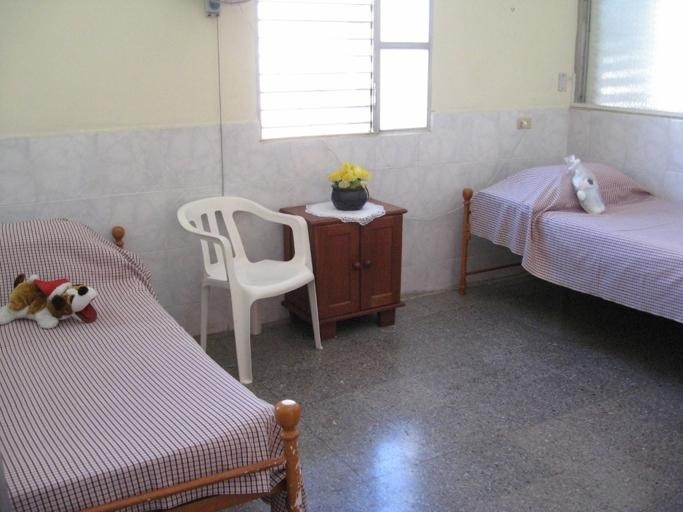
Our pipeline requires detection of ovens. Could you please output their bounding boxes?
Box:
[0,217,305,511]
[456,187,682,337]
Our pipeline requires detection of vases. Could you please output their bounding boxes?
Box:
[327,162,372,190]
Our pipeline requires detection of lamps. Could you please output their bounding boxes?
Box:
[175,195,324,384]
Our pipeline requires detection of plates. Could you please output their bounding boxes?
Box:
[280,199,409,340]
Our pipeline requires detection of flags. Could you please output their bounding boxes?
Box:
[330,186,367,210]
